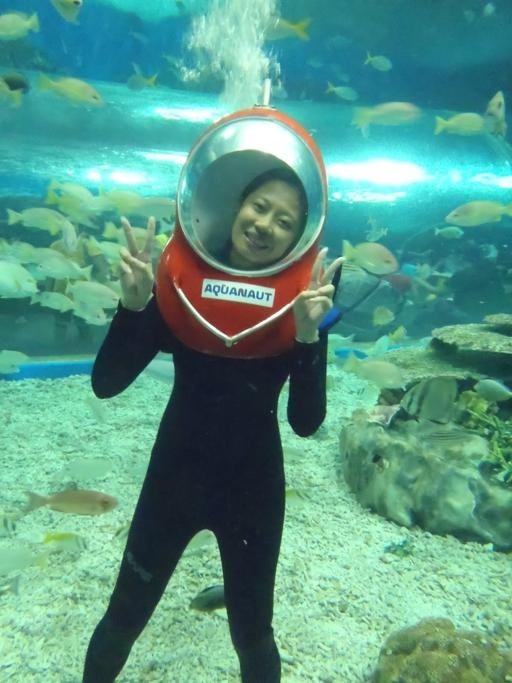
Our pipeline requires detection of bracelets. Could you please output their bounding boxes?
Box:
[117,297,147,320]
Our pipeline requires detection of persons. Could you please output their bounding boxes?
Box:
[83,168,346,682]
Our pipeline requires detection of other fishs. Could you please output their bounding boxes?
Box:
[0,1,512,614]
